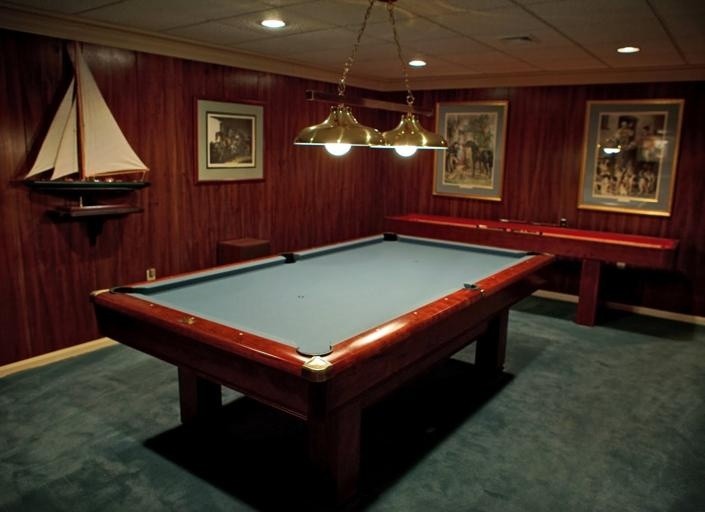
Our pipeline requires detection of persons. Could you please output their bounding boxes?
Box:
[447,141,464,174]
[596,119,658,197]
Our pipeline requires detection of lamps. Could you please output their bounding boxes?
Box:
[288,0,453,160]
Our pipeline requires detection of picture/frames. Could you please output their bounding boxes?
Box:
[189,91,268,188]
[576,97,688,219]
[428,96,510,203]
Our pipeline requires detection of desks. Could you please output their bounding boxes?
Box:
[85,224,560,508]
[386,211,683,329]
